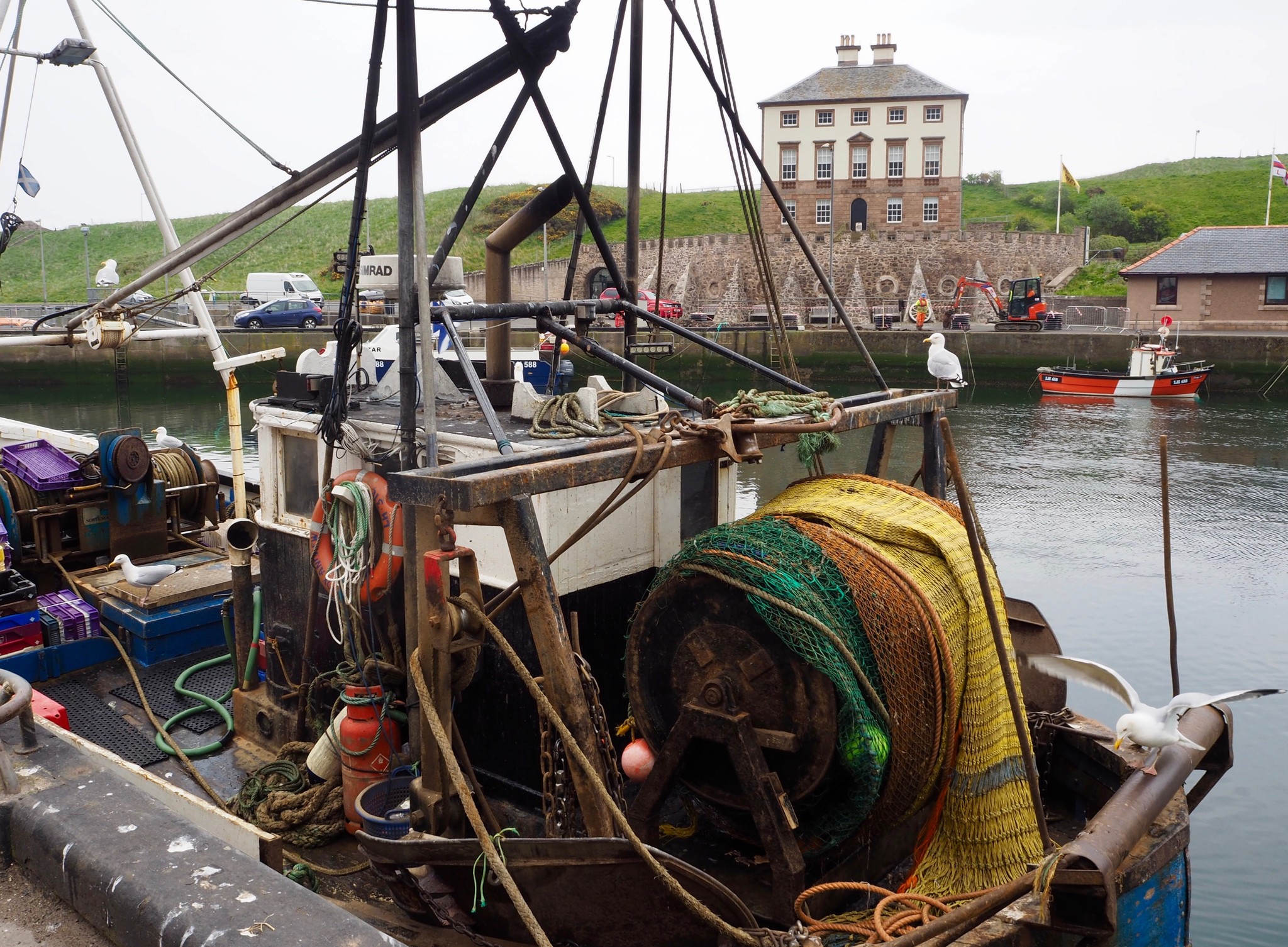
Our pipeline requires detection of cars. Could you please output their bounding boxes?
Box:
[538,325,576,379]
[358,289,386,302]
[239,292,248,304]
[434,285,475,306]
[233,297,325,328]
[113,287,154,304]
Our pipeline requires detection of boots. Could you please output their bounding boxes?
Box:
[916,326,919,331]
[919,325,923,330]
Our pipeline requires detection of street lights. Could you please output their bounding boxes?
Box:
[80,223,91,288]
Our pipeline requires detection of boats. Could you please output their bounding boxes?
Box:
[1037,312,1213,399]
[348,315,573,391]
[0,0,1235,947]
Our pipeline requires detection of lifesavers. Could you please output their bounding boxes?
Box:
[1157,326,1170,337]
[1140,344,1164,351]
[309,470,404,605]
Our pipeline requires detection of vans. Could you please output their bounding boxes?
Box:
[246,272,325,309]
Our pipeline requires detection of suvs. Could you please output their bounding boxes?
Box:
[599,287,683,321]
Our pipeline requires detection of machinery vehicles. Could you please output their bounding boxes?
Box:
[943,276,1048,332]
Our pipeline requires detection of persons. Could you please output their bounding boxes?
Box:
[914,294,929,331]
[592,286,601,299]
[1027,285,1035,298]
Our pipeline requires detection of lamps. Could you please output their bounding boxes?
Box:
[625,333,675,356]
[48,38,95,66]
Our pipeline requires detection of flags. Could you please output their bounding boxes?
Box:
[1273,156,1288,186]
[1061,163,1080,194]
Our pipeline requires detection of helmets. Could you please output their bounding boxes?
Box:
[921,293,926,297]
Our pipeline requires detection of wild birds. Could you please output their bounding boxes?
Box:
[923,333,968,391]
[1028,653,1288,776]
[108,554,185,607]
[151,426,199,453]
[96,259,119,298]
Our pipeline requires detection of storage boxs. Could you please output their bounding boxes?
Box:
[0,569,101,658]
[2,439,84,492]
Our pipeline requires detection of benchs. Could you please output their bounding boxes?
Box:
[748,305,775,322]
[872,305,902,323]
[809,306,838,324]
[699,303,719,315]
